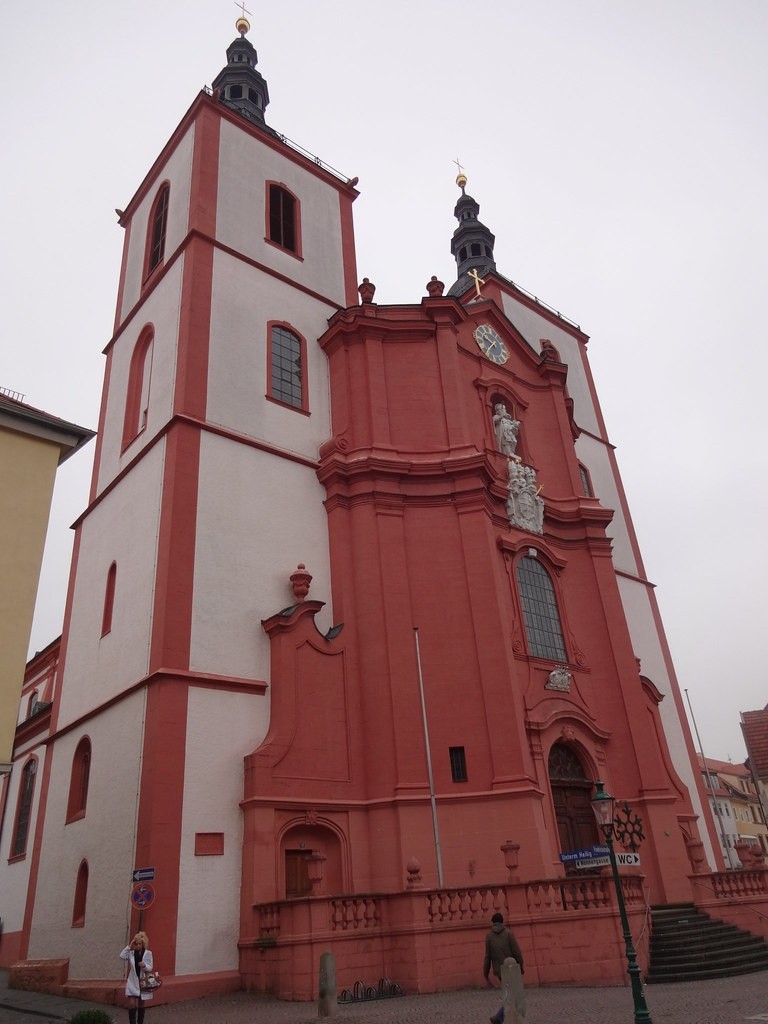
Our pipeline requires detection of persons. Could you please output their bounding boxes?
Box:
[120,932,155,1024]
[507,461,537,492]
[493,404,520,455]
[483,913,526,1024]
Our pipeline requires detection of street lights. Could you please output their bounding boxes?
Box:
[589,780,652,1024]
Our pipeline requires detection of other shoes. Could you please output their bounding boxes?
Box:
[490,1014,501,1024]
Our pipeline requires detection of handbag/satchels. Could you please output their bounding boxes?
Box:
[139,968,163,992]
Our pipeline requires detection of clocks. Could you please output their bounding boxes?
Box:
[473,324,511,366]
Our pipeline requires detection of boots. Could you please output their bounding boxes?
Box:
[137,1007,145,1024]
[128,1006,137,1024]
